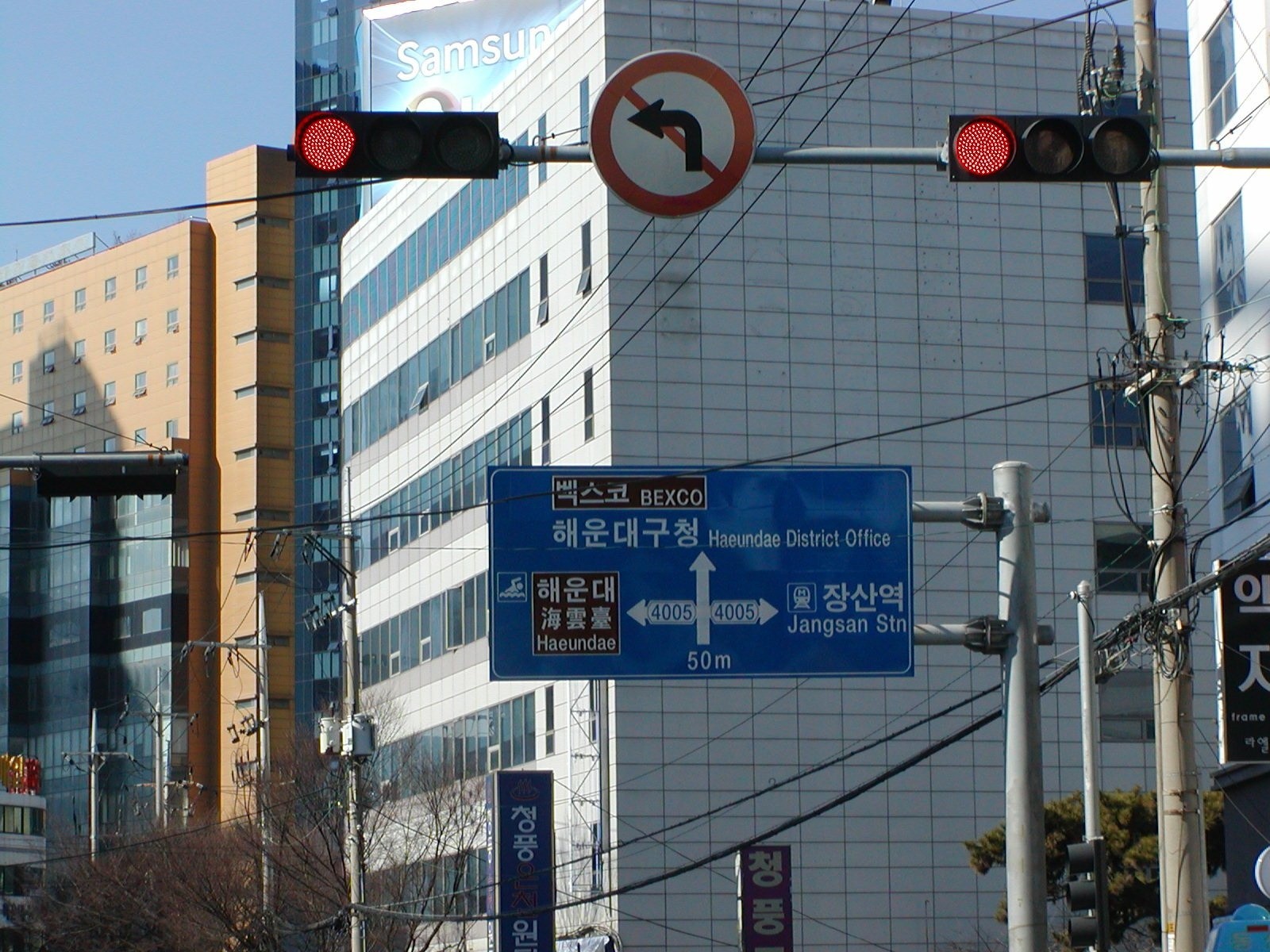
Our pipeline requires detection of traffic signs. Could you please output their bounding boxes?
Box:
[485,463,916,680]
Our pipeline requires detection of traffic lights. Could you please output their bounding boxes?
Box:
[1064,837,1111,952]
[949,114,1153,185]
[293,110,500,181]
